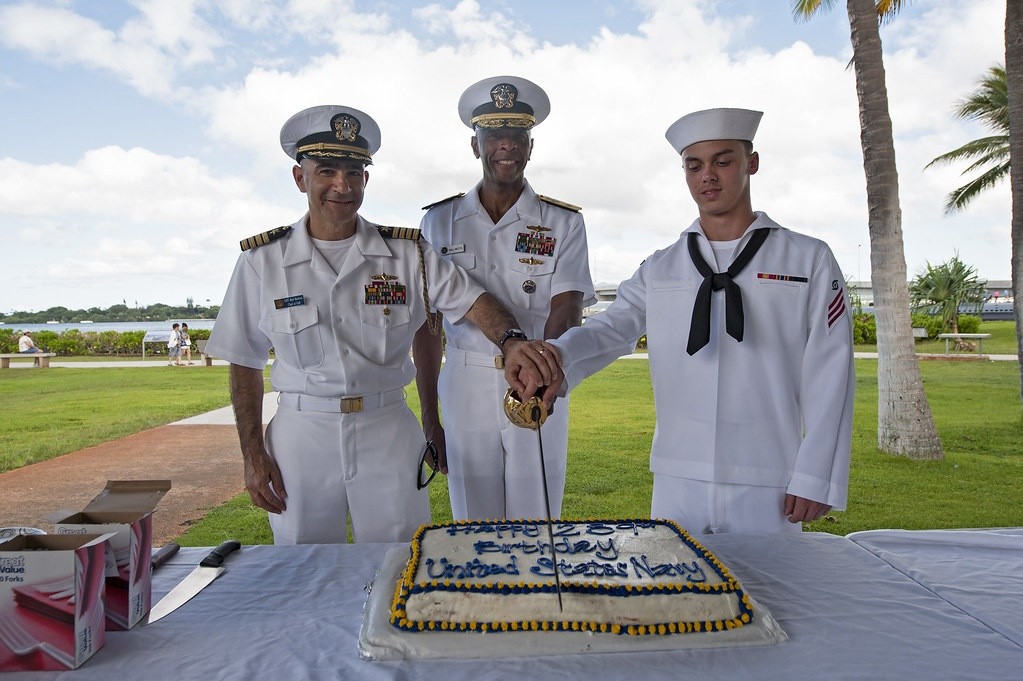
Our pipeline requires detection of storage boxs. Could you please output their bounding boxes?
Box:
[0,528,121,673]
[53,477,173,633]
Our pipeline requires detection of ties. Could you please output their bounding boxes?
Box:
[686,228,770,356]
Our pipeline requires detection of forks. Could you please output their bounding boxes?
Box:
[115,548,130,571]
[33,576,75,604]
[0,613,75,669]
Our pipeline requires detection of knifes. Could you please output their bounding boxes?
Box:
[140,539,241,626]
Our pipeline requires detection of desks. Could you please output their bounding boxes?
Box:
[0,532,1023,681]
[845,506,1023,645]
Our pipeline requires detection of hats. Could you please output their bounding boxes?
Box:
[459,76,550,130]
[666,109,764,154]
[23,329,33,334]
[280,105,381,165]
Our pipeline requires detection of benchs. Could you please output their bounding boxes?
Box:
[0,352,57,369]
[939,333,992,355]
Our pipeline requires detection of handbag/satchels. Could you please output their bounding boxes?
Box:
[185,338,191,346]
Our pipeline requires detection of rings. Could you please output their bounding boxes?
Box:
[539,348,547,355]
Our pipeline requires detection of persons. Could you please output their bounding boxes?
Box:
[517,108,857,533]
[19,328,43,366]
[203,104,563,544]
[175,323,195,365]
[168,322,184,366]
[412,77,598,522]
[994,290,999,303]
[1004,290,1008,299]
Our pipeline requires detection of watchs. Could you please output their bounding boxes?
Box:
[499,329,527,347]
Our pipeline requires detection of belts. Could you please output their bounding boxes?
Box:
[446,347,504,370]
[280,389,404,414]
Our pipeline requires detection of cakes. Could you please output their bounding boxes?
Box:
[390,516,755,636]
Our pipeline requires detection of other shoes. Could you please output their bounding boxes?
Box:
[169,363,173,366]
[188,362,194,366]
[177,363,185,366]
